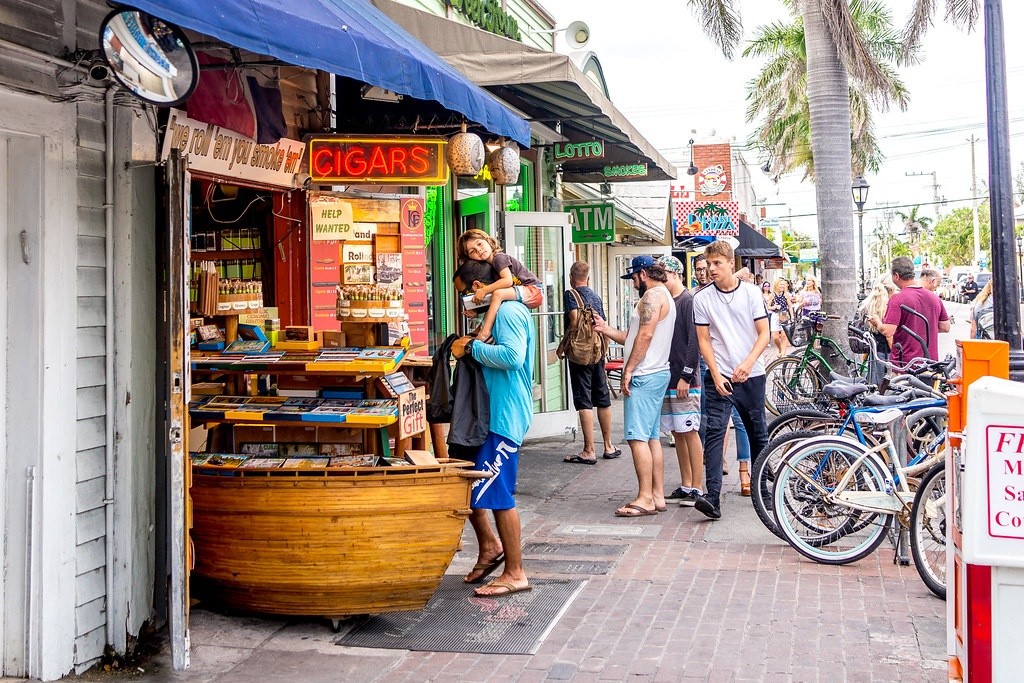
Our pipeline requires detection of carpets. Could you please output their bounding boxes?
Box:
[334,575,588,656]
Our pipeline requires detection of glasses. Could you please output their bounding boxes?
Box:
[763,286,770,288]
[626,266,646,274]
[458,279,482,299]
[696,267,707,274]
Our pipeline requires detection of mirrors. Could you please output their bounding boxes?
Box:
[99,7,199,107]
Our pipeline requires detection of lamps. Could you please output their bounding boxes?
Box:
[361,84,404,103]
[687,138,698,175]
[529,21,590,49]
[730,141,779,183]
[484,138,506,153]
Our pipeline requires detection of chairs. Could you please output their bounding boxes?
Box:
[603,353,625,393]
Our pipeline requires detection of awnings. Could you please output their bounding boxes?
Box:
[734,219,783,257]
[372,0,676,181]
[98,0,532,151]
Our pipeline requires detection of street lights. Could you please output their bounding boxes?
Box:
[851,176,869,300]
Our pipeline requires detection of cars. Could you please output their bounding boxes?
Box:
[939,266,992,303]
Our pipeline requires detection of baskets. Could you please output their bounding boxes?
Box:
[772,373,831,417]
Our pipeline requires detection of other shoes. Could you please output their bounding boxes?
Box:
[722,458,729,475]
[702,451,707,464]
[669,433,676,447]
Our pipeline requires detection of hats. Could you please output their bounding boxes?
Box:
[620,255,657,279]
[655,255,684,273]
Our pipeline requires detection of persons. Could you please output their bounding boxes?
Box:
[964,277,994,340]
[916,268,955,325]
[556,261,622,464]
[694,241,773,519]
[589,256,676,516]
[962,275,978,302]
[867,273,895,384]
[756,274,821,357]
[653,257,704,506]
[451,260,535,596]
[707,267,751,495]
[459,228,543,346]
[691,254,707,465]
[869,256,951,479]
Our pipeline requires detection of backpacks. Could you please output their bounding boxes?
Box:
[563,290,605,365]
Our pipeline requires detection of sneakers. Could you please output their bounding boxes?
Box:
[694,495,721,518]
[664,488,689,504]
[680,489,704,506]
[760,486,773,510]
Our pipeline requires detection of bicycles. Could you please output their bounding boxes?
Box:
[751,309,959,599]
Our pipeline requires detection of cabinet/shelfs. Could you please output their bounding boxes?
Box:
[189,360,421,458]
[190,250,262,312]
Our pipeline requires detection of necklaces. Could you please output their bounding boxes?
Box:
[716,275,735,304]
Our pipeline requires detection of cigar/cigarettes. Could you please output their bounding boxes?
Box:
[196,258,262,317]
[336,285,404,300]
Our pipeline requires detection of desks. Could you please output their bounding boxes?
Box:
[607,344,625,393]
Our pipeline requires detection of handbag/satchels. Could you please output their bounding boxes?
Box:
[779,315,789,326]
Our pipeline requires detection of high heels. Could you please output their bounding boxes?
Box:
[739,469,752,496]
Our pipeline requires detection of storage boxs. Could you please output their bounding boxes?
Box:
[190,318,407,372]
[190,370,415,425]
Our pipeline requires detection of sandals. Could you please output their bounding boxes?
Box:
[468,332,478,337]
[482,334,495,345]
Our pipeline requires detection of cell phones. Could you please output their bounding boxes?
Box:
[724,382,732,392]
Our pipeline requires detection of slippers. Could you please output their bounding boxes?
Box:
[655,505,667,512]
[466,555,505,583]
[475,577,533,597]
[603,446,622,458]
[563,454,597,464]
[615,503,659,516]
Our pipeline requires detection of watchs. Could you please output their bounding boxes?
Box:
[464,339,475,355]
